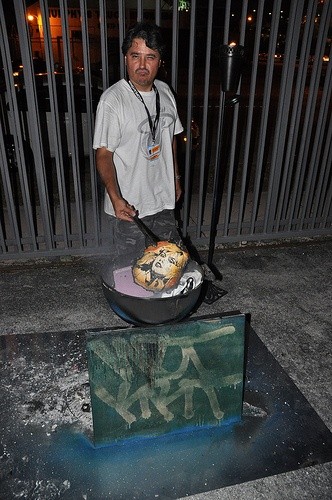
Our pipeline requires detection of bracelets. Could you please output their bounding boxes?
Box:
[175,176,180,178]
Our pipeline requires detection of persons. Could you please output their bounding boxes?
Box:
[93,21,188,271]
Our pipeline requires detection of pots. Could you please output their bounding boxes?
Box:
[100,254,202,326]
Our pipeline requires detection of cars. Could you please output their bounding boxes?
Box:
[0,81,208,175]
